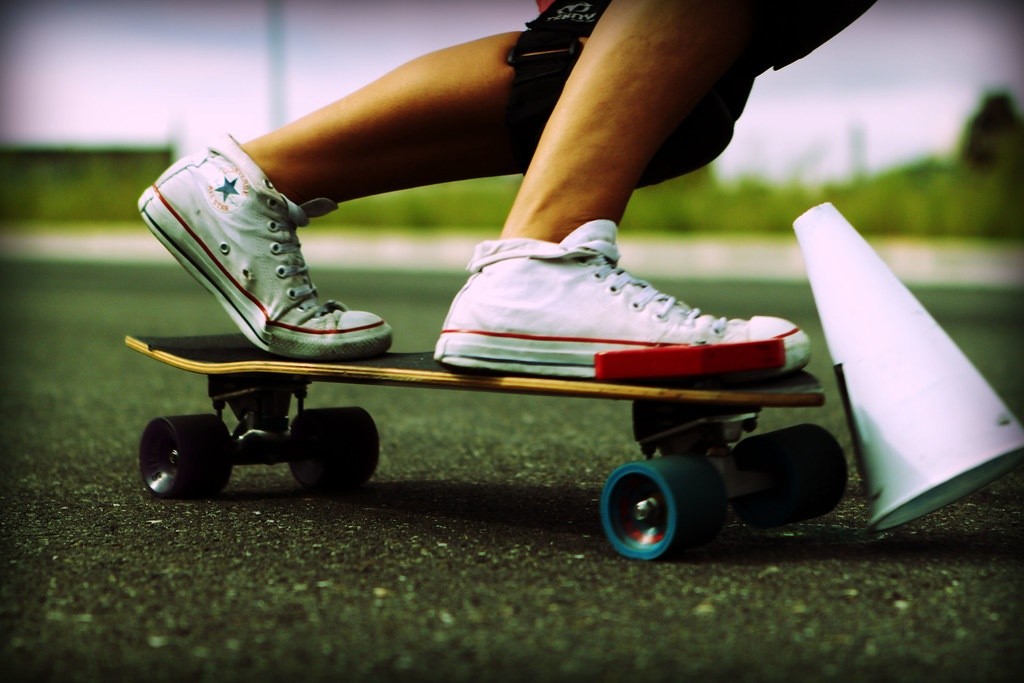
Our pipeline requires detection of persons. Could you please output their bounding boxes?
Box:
[130,0,905,388]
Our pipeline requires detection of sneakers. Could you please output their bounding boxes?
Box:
[137,132,394,361]
[432,218,812,382]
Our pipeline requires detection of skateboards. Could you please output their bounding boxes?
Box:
[124,330,849,559]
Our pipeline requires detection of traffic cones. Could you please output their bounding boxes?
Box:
[791,200,1023,533]
